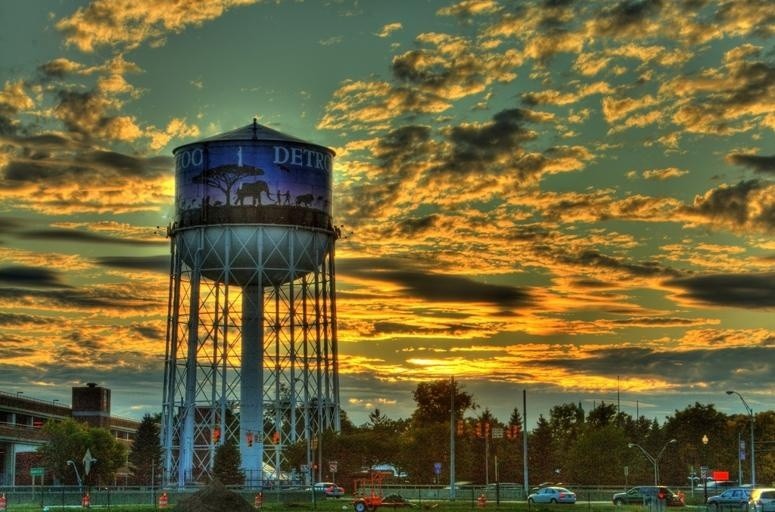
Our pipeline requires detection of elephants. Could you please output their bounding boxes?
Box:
[233,179,275,206]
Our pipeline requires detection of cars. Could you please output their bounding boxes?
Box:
[748,487,775,512]
[304,481,345,500]
[527,487,577,505]
[612,486,684,506]
[706,487,752,512]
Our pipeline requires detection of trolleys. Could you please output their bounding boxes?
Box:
[350,478,413,512]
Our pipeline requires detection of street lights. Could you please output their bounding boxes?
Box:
[725,390,757,486]
[627,439,678,486]
